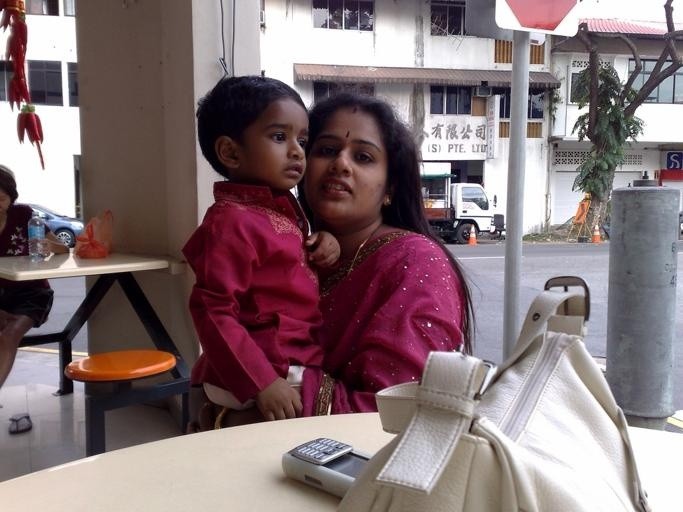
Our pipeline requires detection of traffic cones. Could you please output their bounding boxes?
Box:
[468,224,477,246]
[591,225,600,242]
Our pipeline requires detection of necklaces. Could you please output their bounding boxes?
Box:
[345,233,375,279]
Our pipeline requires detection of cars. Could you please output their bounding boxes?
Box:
[17,204,85,248]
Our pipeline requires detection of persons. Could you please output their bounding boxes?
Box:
[0,162,69,410]
[178,75,342,423]
[186,85,481,434]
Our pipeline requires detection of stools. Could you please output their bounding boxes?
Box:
[62,348,177,462]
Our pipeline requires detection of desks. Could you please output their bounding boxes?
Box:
[1,244,191,434]
[1,411,683,510]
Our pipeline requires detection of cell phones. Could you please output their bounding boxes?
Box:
[283,435,372,500]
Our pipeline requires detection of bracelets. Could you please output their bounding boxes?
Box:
[214,406,227,432]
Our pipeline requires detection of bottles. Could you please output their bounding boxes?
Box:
[27,211,46,264]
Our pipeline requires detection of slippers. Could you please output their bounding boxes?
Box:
[8,412,32,433]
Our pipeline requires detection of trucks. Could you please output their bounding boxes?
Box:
[419,173,507,245]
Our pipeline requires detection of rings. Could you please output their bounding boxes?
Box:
[42,253,47,257]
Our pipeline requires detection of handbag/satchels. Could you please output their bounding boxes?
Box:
[336,276,650,511]
